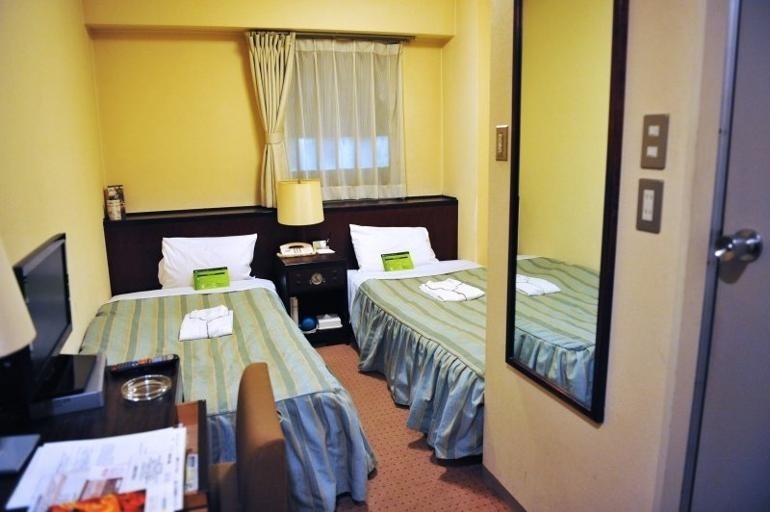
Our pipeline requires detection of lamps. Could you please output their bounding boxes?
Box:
[277,179,326,226]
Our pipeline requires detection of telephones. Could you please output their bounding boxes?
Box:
[279,241,315,257]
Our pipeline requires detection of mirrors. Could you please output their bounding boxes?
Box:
[503,0,629,425]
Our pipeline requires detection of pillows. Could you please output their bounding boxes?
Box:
[158,234,257,291]
[349,224,439,272]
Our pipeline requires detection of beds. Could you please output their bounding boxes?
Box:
[346,221,486,460]
[515,256,599,412]
[80,237,378,511]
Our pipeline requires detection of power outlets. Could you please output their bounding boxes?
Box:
[634,176,664,234]
[494,125,508,163]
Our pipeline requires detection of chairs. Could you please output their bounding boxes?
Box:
[213,362,293,511]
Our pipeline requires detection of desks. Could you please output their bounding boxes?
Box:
[0,354,205,512]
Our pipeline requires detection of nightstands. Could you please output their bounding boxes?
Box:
[275,244,352,345]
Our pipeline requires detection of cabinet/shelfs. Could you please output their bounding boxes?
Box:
[103,194,458,346]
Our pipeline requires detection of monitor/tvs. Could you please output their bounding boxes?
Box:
[14,231,98,401]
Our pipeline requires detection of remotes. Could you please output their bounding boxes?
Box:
[109,352,180,377]
